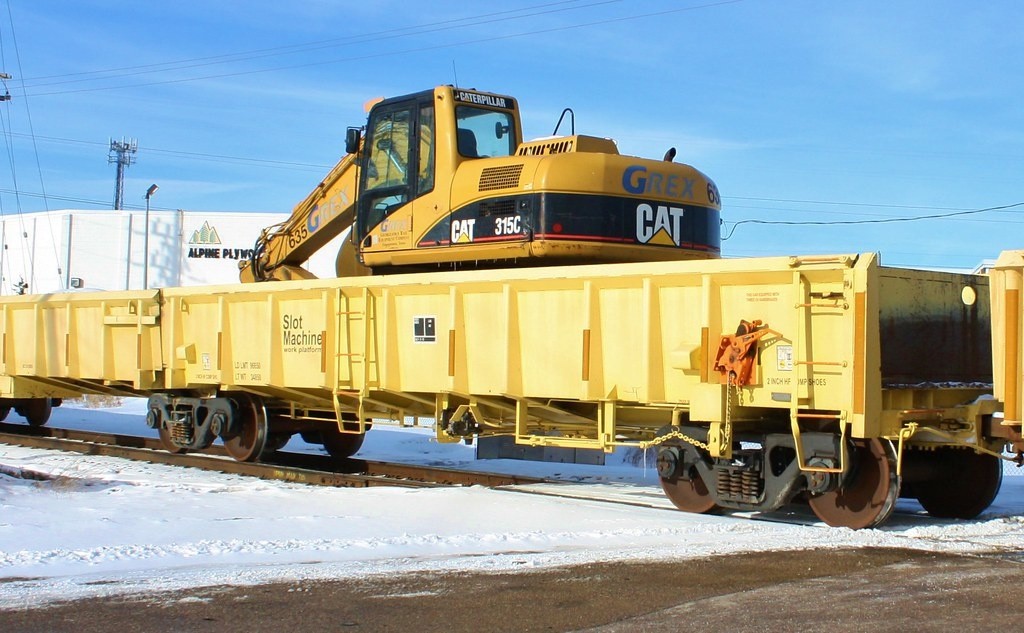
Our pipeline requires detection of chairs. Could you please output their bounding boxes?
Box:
[456,128,477,158]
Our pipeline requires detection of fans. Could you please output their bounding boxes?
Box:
[496,122,509,138]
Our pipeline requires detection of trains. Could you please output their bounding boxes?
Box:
[1,249,1023,531]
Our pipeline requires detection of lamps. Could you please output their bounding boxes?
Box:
[71,278,80,287]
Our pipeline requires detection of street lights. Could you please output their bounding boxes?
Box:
[144,184,160,290]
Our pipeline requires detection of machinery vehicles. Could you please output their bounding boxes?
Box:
[237,84,722,283]
